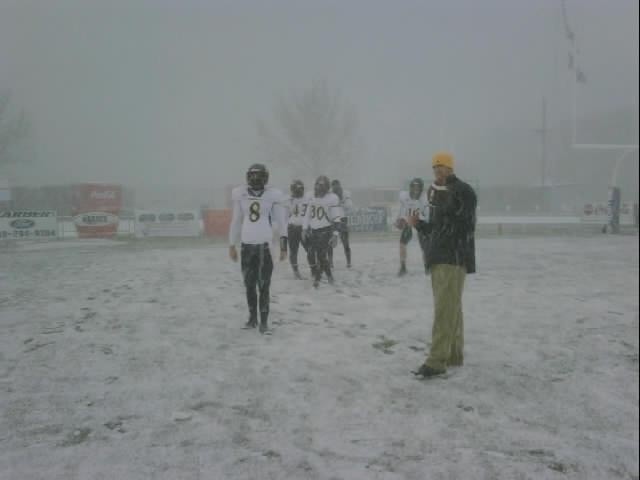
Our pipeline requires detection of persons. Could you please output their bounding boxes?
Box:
[286,180,317,280]
[301,175,341,286]
[327,179,352,269]
[227,161,292,336]
[409,152,477,381]
[609,185,621,234]
[392,177,430,278]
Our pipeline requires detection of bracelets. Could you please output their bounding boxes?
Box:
[280,235,288,251]
[332,230,339,235]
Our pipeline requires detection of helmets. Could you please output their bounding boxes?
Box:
[432,153,453,169]
[314,176,330,197]
[247,163,269,188]
[409,178,424,199]
[290,180,304,197]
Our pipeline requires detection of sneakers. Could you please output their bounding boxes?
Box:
[293,270,334,287]
[397,265,407,276]
[411,363,448,378]
[259,320,272,334]
[241,318,258,329]
